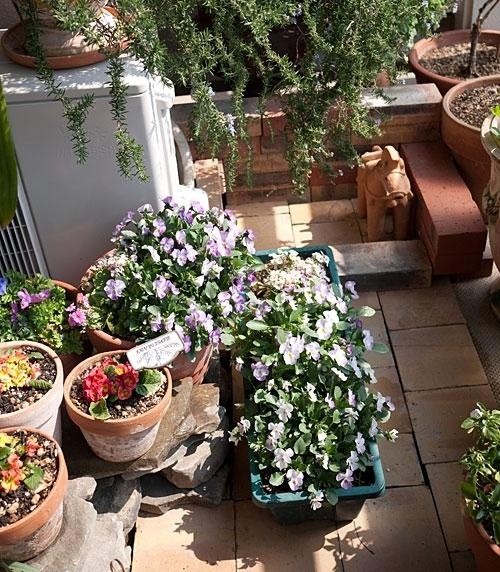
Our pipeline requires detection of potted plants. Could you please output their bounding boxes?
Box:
[460,402,500,572]
[0,0,449,197]
[480,105,499,319]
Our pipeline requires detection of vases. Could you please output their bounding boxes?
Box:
[244,245,386,525]
[82,248,212,385]
[0,426,68,562]
[0,341,64,448]
[409,27,500,95]
[64,350,172,463]
[50,279,93,380]
[442,74,500,224]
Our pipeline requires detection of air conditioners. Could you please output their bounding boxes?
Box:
[0,54,193,287]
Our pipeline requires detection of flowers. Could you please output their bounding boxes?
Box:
[0,346,53,394]
[0,270,103,356]
[229,251,399,511]
[90,196,272,362]
[0,433,44,493]
[82,357,166,420]
